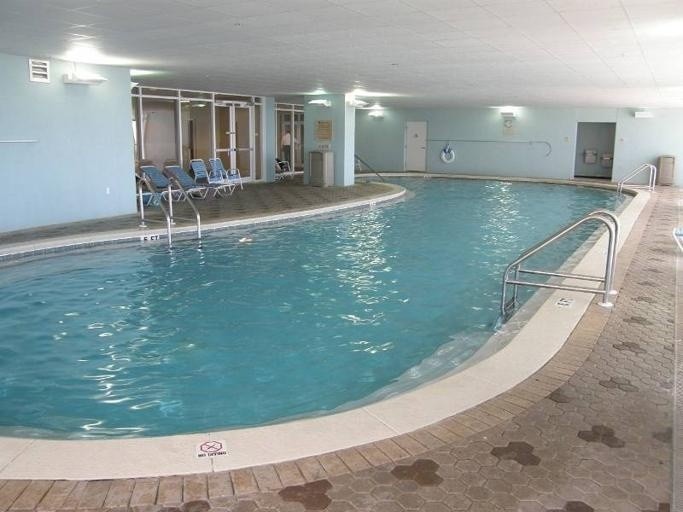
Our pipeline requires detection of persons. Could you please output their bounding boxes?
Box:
[280,130,300,168]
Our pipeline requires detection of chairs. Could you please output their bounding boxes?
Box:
[139,158,244,207]
[272,160,294,182]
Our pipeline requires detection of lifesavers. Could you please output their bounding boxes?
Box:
[441,148,454,162]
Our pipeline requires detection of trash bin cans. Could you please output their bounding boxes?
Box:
[309,151,335,188]
[655,155,675,186]
